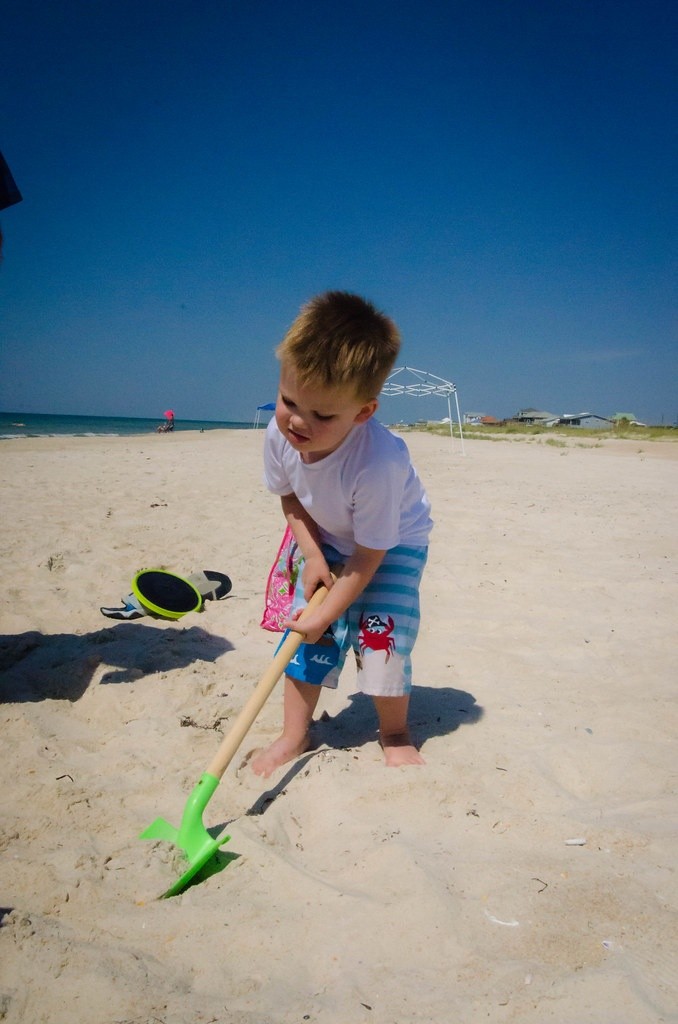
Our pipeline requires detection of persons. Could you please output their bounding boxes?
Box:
[169,414,175,431]
[249,290,434,777]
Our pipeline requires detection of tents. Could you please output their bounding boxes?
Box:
[252,402,277,429]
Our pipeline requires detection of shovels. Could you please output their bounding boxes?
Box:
[140,563,345,899]
